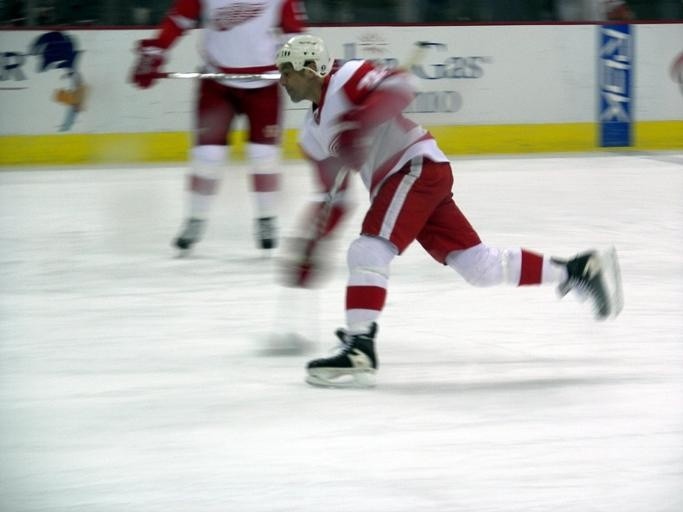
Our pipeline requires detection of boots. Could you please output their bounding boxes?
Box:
[175,217,205,249]
[257,219,277,249]
[306,323,378,377]
[551,249,613,323]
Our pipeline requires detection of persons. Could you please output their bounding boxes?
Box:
[128,1,306,251]
[0,0,672,32]
[274,34,614,378]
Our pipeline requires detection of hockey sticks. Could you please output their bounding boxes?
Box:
[153,41,429,79]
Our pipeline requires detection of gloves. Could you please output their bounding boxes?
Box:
[327,110,368,170]
[125,39,165,91]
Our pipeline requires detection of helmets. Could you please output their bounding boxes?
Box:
[275,36,334,78]
